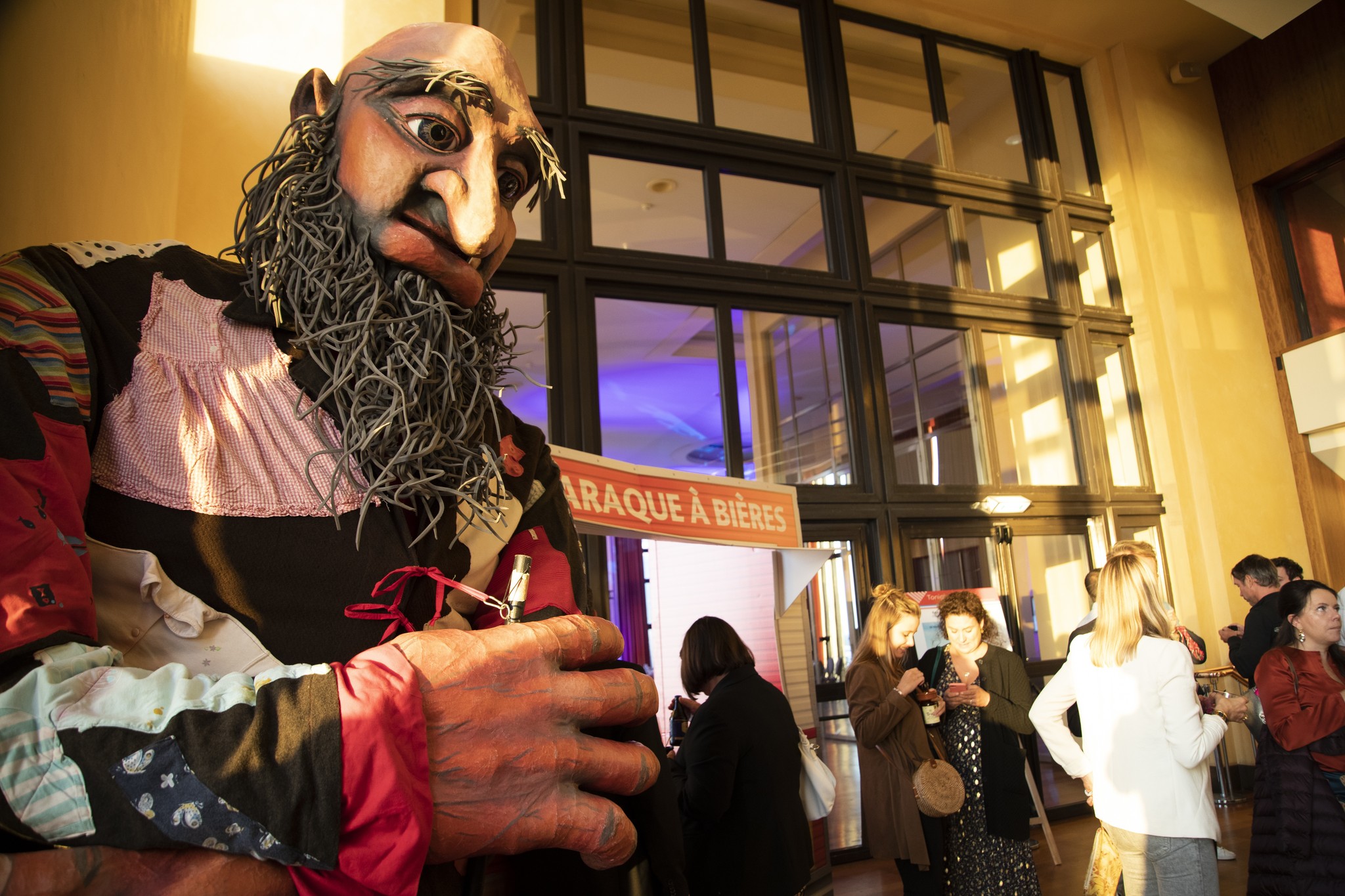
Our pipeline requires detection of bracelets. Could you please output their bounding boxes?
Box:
[1209,710,1229,726]
[893,686,906,699]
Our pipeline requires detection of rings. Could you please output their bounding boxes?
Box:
[970,698,973,704]
[1242,713,1248,720]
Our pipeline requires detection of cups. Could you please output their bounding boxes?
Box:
[917,690,942,727]
[1210,689,1241,709]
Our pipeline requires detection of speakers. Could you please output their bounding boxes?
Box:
[1169,62,1202,84]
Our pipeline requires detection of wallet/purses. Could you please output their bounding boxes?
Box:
[1083,820,1122,896]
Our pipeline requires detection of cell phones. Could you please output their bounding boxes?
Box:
[949,683,968,695]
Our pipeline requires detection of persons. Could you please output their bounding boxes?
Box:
[1067,538,1207,737]
[1029,553,1250,895]
[1217,554,1284,689]
[2,21,661,894]
[846,582,950,896]
[666,617,815,895]
[1269,558,1303,589]
[916,592,1044,896]
[1246,580,1345,896]
[1074,567,1179,627]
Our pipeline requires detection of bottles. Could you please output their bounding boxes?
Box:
[669,695,689,746]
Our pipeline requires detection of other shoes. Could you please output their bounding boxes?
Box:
[1217,846,1237,860]
[1028,837,1039,849]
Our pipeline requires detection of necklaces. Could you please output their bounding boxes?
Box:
[963,671,971,678]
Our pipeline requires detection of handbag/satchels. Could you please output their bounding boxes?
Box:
[913,758,965,818]
[798,725,836,821]
[1239,655,1298,744]
